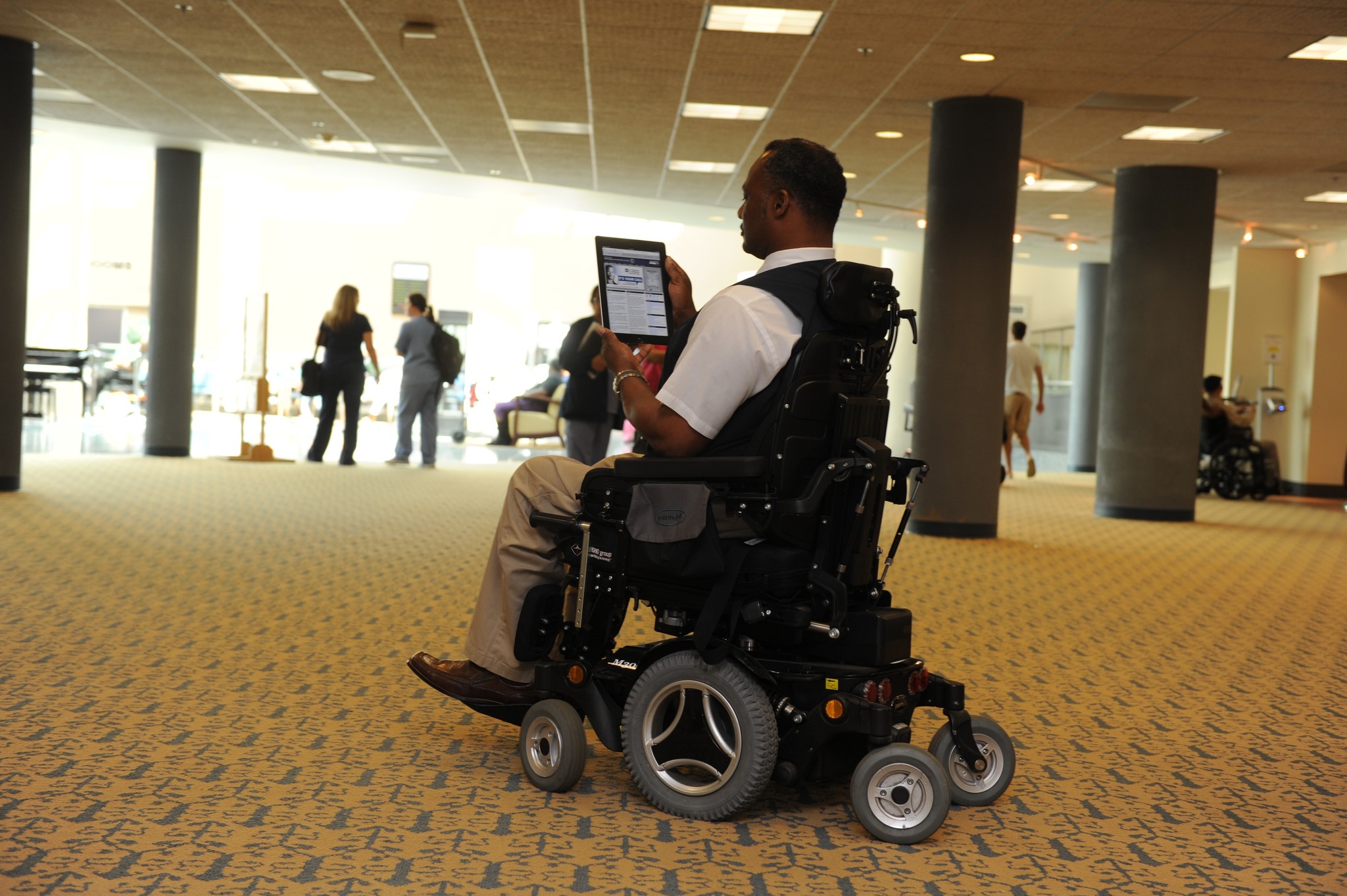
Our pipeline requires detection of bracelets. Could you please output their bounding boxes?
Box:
[612,370,650,401]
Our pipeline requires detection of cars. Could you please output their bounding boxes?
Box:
[435,384,469,443]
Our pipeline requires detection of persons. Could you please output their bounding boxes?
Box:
[559,285,619,464]
[489,358,560,445]
[1004,322,1046,479]
[384,293,443,469]
[606,265,617,284]
[403,138,849,707]
[307,286,382,466]
[1201,374,1283,501]
[85,340,149,407]
[632,341,669,457]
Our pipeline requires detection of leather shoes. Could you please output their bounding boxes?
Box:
[406,651,547,708]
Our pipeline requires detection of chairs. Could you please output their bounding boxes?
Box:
[506,382,569,451]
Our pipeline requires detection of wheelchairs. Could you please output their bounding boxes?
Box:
[1199,398,1276,504]
[508,252,1022,846]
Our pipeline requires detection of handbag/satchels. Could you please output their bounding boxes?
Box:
[301,334,326,397]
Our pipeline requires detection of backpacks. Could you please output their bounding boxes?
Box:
[430,320,465,384]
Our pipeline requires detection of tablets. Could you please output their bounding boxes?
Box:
[594,235,674,346]
